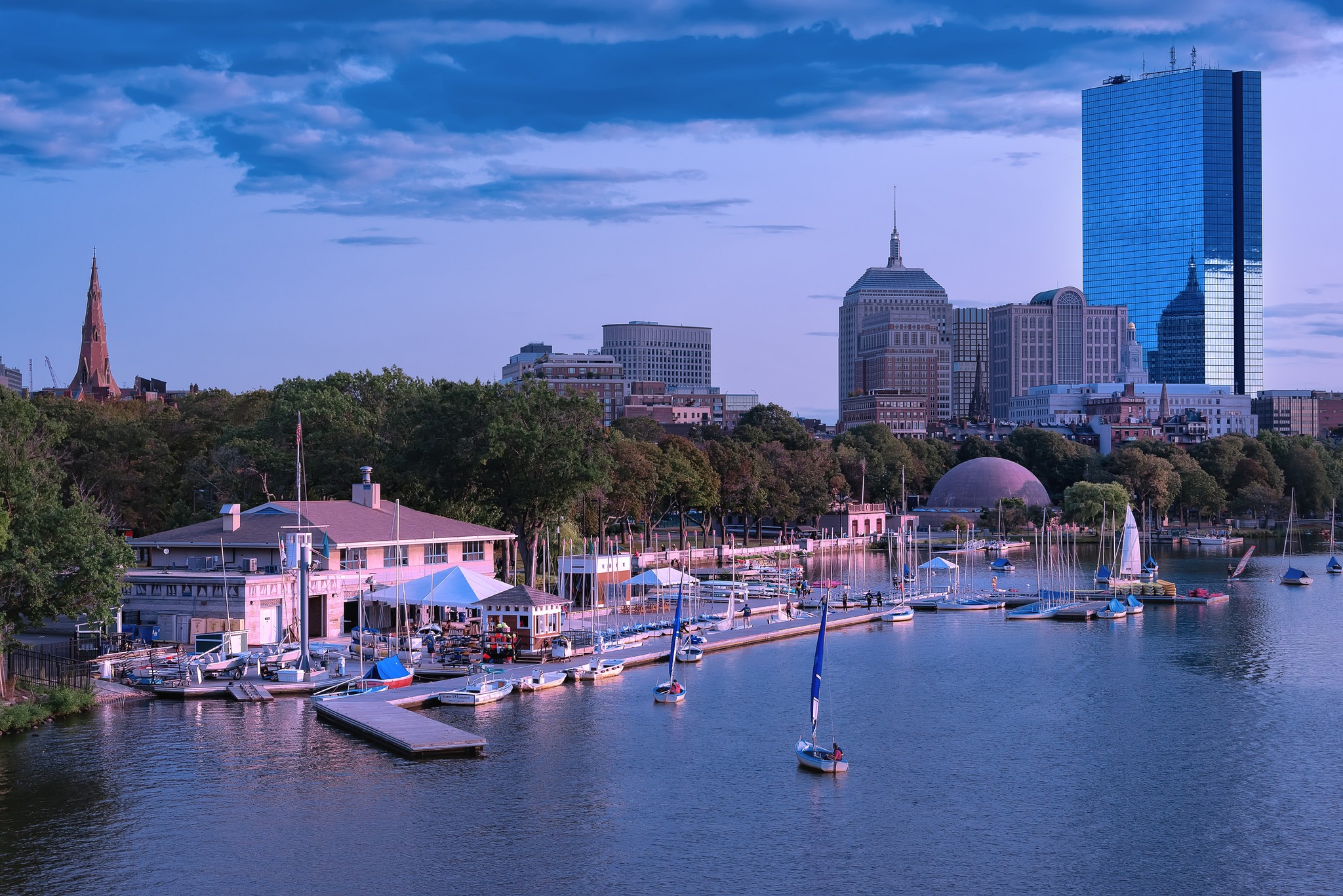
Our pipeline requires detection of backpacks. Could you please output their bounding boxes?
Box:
[801,583,805,589]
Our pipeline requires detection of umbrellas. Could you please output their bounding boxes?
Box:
[607,545,627,554]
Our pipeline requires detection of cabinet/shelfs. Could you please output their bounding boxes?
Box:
[483,631,518,663]
[621,605,647,615]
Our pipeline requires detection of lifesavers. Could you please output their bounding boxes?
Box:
[520,621,524,627]
[601,643,606,652]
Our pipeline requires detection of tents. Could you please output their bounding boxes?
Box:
[917,556,960,594]
[363,566,515,646]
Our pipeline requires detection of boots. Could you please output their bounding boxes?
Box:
[876,606,878,612]
[881,607,884,611]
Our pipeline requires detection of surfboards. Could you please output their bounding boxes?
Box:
[1224,578,1240,581]
[750,567,792,570]
[738,571,810,598]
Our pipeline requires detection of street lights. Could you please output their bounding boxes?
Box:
[1178,486,1182,532]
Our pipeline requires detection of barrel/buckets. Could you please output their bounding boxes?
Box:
[507,657,512,663]
[320,660,330,673]
[491,636,517,643]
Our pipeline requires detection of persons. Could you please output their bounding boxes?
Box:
[736,573,999,629]
[926,528,929,537]
[728,539,736,549]
[445,619,454,633]
[660,544,678,552]
[519,618,524,627]
[1121,574,1136,580]
[908,529,910,534]
[990,533,1028,546]
[1227,564,1237,579]
[777,531,880,546]
[822,743,844,762]
[407,608,414,633]
[422,631,436,664]
[562,604,570,620]
[953,530,956,535]
[669,679,682,695]
[673,558,682,572]
[633,549,639,557]
[416,609,423,630]
[493,555,515,571]
[1079,510,1339,543]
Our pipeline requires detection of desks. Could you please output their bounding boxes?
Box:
[683,597,705,608]
[634,603,657,613]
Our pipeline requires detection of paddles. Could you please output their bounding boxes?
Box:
[435,636,481,663]
[1324,538,1337,541]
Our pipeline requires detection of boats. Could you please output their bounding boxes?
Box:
[509,666,567,692]
[438,662,514,706]
[1320,529,1340,543]
[564,656,627,680]
[1179,534,1244,545]
[1304,533,1311,534]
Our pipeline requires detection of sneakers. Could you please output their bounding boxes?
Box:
[431,661,435,664]
[429,661,431,664]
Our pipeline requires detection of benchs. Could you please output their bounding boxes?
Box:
[620,602,681,615]
[646,602,669,612]
[682,599,707,609]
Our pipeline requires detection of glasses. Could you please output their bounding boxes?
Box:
[843,592,845,592]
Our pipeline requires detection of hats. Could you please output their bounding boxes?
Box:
[895,574,897,577]
[429,631,435,635]
[995,576,997,579]
[1129,575,1131,577]
[672,682,675,686]
[823,594,827,596]
[673,679,677,683]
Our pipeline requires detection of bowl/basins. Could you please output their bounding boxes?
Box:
[111,677,119,682]
[484,658,491,663]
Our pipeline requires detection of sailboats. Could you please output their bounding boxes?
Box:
[881,533,917,622]
[513,497,1177,655]
[794,574,849,773]
[675,542,702,663]
[125,514,443,704]
[1279,487,1314,585]
[1223,545,1255,581]
[652,555,687,704]
[1325,498,1343,573]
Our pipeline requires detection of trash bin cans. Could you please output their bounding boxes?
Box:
[983,529,989,536]
[921,527,925,532]
[121,623,137,640]
[1115,531,1121,536]
[925,527,928,532]
[449,611,458,622]
[459,612,466,622]
[917,525,922,532]
[136,624,162,645]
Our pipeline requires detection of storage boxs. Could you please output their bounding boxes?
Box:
[277,669,305,682]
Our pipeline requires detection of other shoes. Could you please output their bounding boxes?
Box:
[827,617,829,618]
[867,610,871,612]
[803,596,806,599]
[798,597,801,599]
[680,646,683,648]
[846,612,847,613]
[843,611,845,613]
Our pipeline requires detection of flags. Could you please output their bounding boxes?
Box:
[296,414,300,445]
[321,530,329,559]
[280,533,287,565]
[589,539,594,556]
